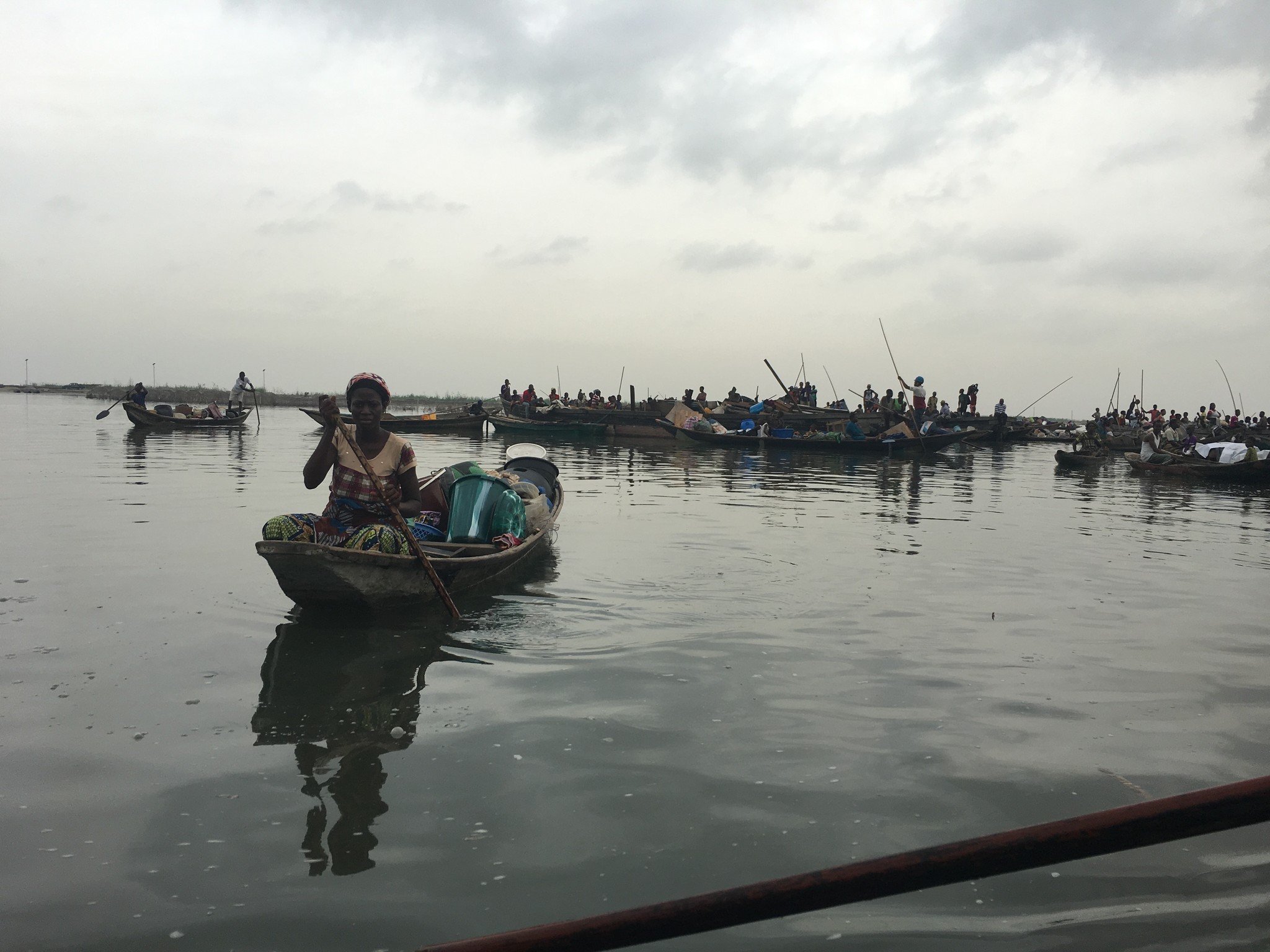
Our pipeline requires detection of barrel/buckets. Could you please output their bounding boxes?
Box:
[1037,432,1045,438]
[706,417,719,424]
[504,456,559,489]
[499,468,553,500]
[446,473,511,546]
[967,426,975,431]
[1028,417,1035,423]
[741,419,755,430]
[506,443,547,466]
[1042,421,1047,426]
[153,405,173,417]
[147,410,157,413]
[218,409,227,416]
[750,400,766,414]
[953,425,961,432]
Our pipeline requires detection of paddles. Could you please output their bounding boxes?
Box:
[95,382,143,420]
[421,772,1270,951]
[319,392,462,620]
[252,388,261,425]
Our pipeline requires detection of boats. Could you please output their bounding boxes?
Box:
[1091,414,1270,476]
[1051,449,1108,468]
[122,401,255,431]
[252,454,564,611]
[486,399,1081,452]
[297,406,503,431]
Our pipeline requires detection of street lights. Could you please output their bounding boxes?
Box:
[262,369,266,393]
[25,359,28,385]
[152,363,156,387]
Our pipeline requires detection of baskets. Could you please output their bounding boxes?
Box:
[411,521,446,543]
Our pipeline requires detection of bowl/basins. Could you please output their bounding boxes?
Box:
[771,429,794,438]
[176,406,193,415]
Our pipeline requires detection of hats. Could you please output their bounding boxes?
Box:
[345,373,391,409]
[976,412,980,415]
[551,387,555,390]
[940,400,945,403]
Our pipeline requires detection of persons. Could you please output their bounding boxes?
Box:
[1230,409,1241,427]
[1067,395,1270,455]
[1206,403,1222,420]
[750,381,981,420]
[501,379,659,428]
[682,389,692,400]
[1244,436,1258,462]
[261,372,422,556]
[1141,404,1160,422]
[457,399,489,420]
[227,372,255,409]
[696,386,707,402]
[1140,419,1173,465]
[126,382,148,410]
[1072,420,1101,454]
[846,412,880,441]
[898,376,926,437]
[994,399,1008,426]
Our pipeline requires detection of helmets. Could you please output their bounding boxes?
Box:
[914,376,924,384]
